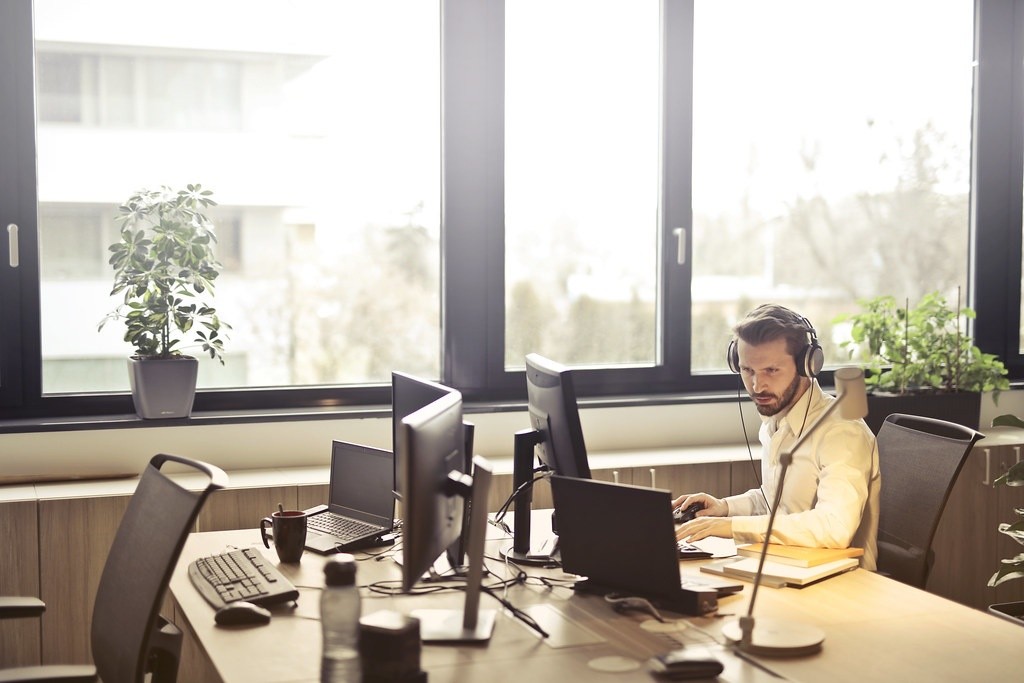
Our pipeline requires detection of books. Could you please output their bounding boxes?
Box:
[699,543,865,589]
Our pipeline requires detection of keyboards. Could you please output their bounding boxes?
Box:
[188,547,299,609]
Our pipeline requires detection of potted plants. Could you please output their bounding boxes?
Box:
[832,292,1011,439]
[93,181,235,420]
[989,412,1024,627]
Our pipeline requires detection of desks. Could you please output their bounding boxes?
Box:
[155,509,1024,683]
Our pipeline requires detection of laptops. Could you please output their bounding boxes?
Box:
[304,440,394,555]
[551,475,744,606]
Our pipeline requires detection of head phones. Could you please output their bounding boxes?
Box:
[727,315,824,377]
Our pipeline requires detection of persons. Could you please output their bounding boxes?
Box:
[671,303,882,573]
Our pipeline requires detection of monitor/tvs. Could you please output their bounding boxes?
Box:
[390,371,475,627]
[499,352,592,563]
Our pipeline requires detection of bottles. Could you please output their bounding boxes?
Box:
[320,555,361,660]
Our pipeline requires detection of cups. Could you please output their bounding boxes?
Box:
[260,511,307,562]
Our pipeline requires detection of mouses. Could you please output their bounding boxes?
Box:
[214,601,271,624]
[672,502,705,523]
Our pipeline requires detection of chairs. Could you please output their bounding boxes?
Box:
[871,411,986,591]
[0,452,231,683]
